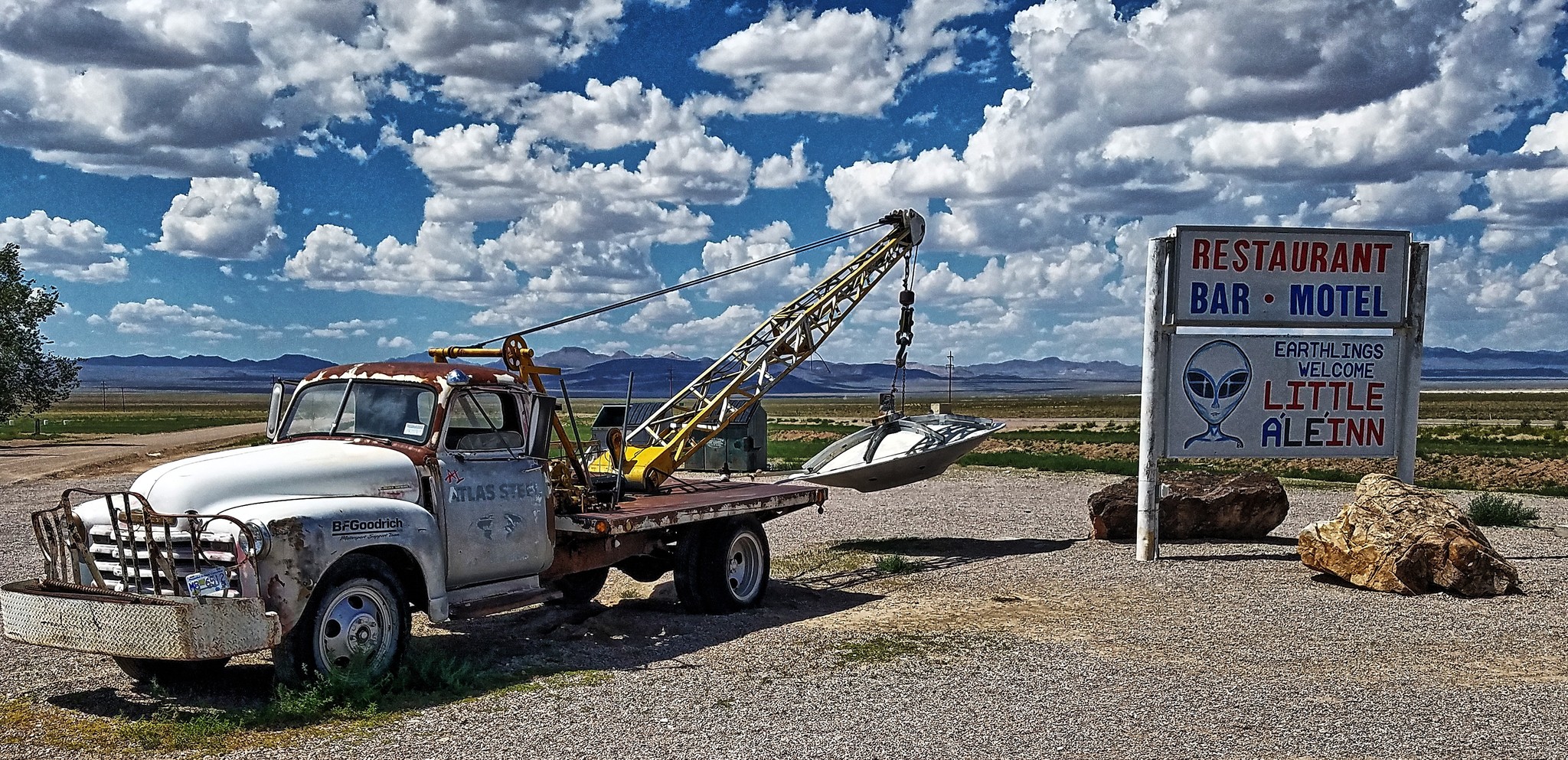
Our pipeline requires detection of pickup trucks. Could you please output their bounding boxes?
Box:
[0,359,831,717]
